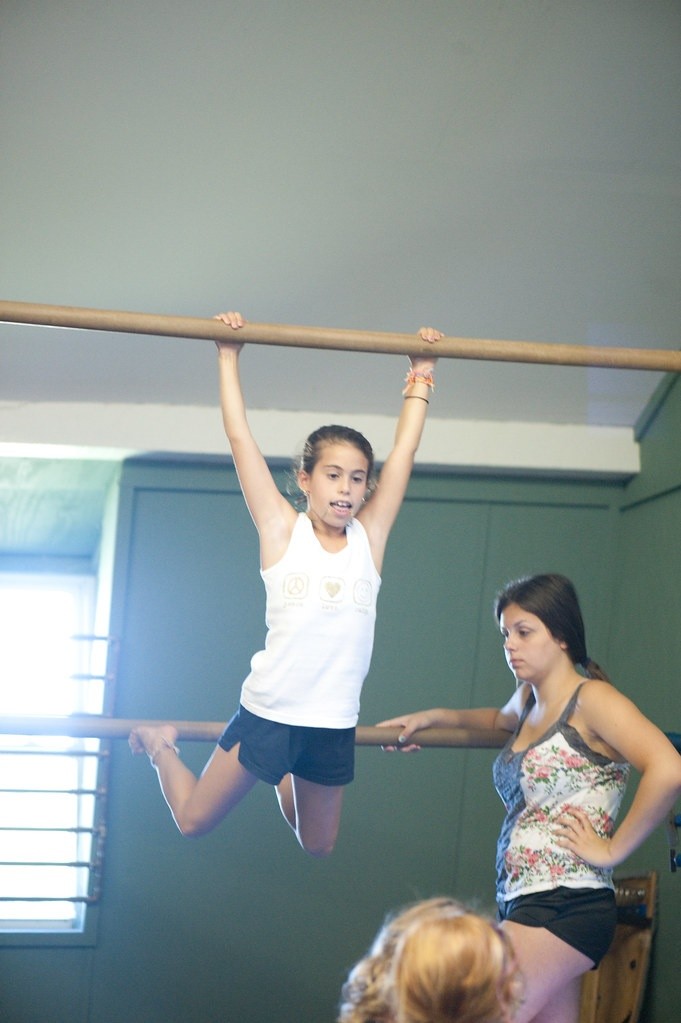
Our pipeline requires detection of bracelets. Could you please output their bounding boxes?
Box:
[406,370,434,386]
[404,395,429,404]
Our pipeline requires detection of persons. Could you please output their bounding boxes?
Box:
[338,897,525,1023]
[130,310,444,855]
[373,573,681,1023]
